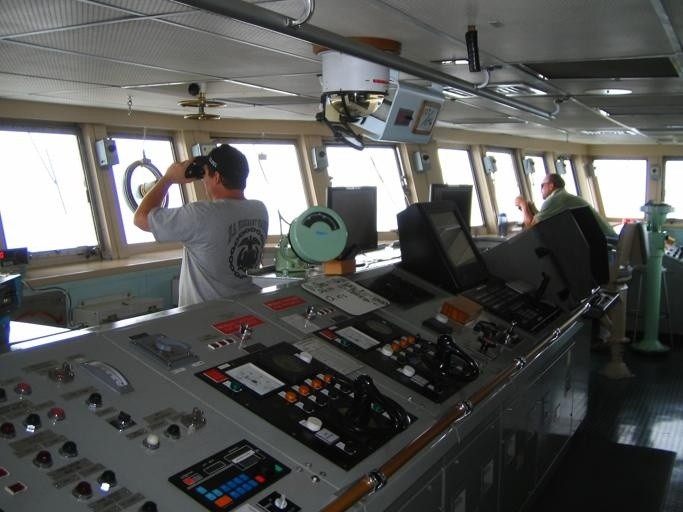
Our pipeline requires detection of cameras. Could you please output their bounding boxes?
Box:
[185,162,205,180]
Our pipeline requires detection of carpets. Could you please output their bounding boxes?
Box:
[526,439,676,511]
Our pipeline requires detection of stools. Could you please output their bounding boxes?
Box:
[628,263,675,351]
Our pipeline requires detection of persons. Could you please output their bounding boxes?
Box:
[514,172,621,255]
[131,140,270,308]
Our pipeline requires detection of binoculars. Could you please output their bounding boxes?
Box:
[185,156,206,180]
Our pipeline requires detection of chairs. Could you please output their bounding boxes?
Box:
[599,221,640,380]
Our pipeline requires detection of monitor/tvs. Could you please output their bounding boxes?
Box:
[325,186,378,260]
[425,207,479,269]
[429,183,473,226]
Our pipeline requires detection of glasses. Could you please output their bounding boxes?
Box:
[541,183,549,188]
